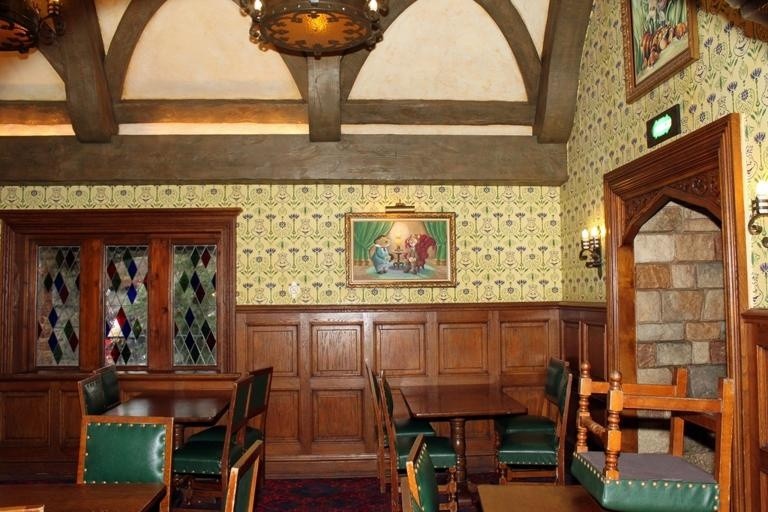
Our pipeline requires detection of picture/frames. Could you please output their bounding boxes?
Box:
[344,212,457,289]
[619,0,701,103]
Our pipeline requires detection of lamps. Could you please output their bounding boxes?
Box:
[234,0,389,59]
[579,227,602,278]
[0,0,63,54]
[747,180,768,250]
